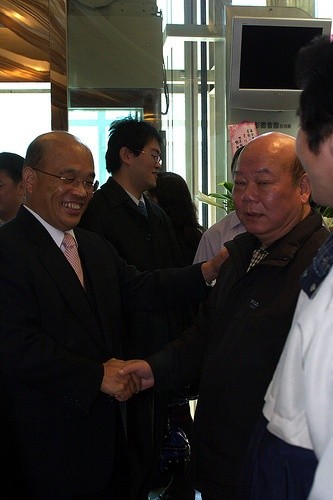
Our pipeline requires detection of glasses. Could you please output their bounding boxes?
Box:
[142,151,163,165]
[35,167,99,194]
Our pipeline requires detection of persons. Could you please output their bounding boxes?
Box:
[0,132,231,500]
[0,34,333,500]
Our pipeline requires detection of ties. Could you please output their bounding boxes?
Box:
[138,201,147,216]
[63,233,85,291]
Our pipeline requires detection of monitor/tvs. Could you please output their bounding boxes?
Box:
[229,16,332,112]
